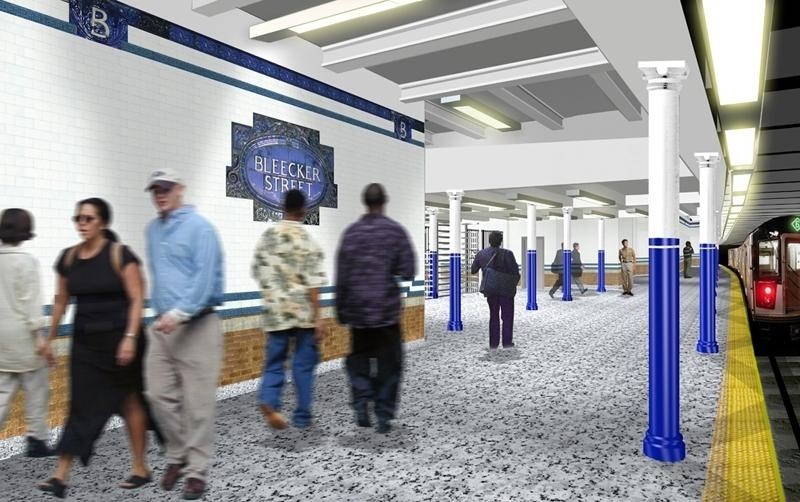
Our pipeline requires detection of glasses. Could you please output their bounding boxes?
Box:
[71,214,96,226]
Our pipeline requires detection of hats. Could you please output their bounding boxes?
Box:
[144,169,186,190]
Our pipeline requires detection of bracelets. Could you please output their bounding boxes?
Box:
[123,333,140,338]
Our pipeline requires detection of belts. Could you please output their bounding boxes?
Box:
[154,308,214,323]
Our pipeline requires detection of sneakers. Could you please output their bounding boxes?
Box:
[356,407,372,429]
[375,404,392,436]
[547,286,638,299]
[27,439,58,458]
[260,403,288,432]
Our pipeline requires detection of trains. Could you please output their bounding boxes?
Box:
[724,208,799,333]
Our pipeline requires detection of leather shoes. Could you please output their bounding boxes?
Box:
[183,475,205,498]
[162,461,181,491]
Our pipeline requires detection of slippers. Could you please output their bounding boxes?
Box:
[37,477,66,498]
[120,472,152,490]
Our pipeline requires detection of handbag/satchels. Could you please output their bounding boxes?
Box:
[479,269,519,299]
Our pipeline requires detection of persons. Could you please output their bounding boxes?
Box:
[471,231,520,350]
[141,170,224,500]
[619,239,636,296]
[571,243,588,294]
[335,183,419,434]
[549,243,563,298]
[0,209,58,457]
[36,198,164,499]
[251,190,329,429]
[683,241,694,278]
